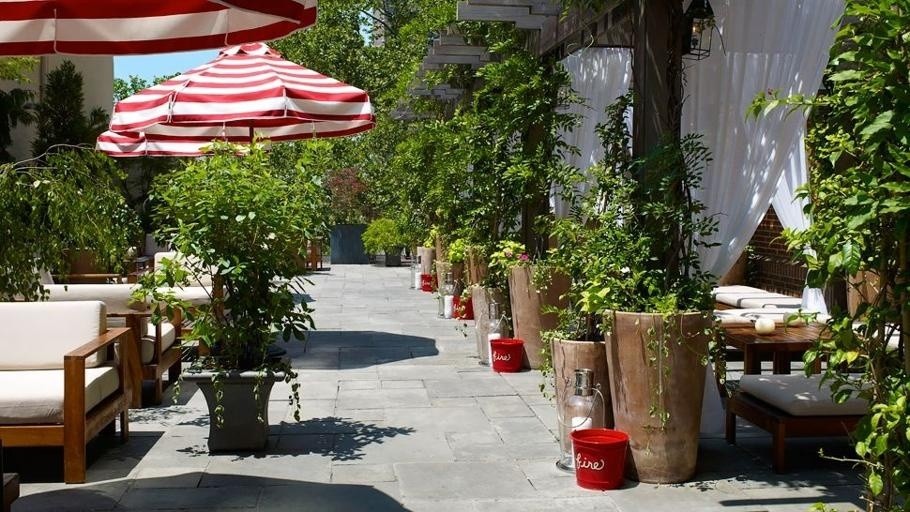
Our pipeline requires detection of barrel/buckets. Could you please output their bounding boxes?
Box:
[490,339,524,371]
[421,274,438,291]
[568,428,629,490]
[453,296,474,320]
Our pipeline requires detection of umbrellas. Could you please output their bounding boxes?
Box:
[109,41,376,154]
[0,0,319,62]
[96,128,278,156]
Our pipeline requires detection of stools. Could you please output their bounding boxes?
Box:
[723,373,873,474]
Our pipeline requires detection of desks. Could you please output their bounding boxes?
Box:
[720,321,838,375]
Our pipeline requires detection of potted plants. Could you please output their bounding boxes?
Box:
[126,131,334,456]
